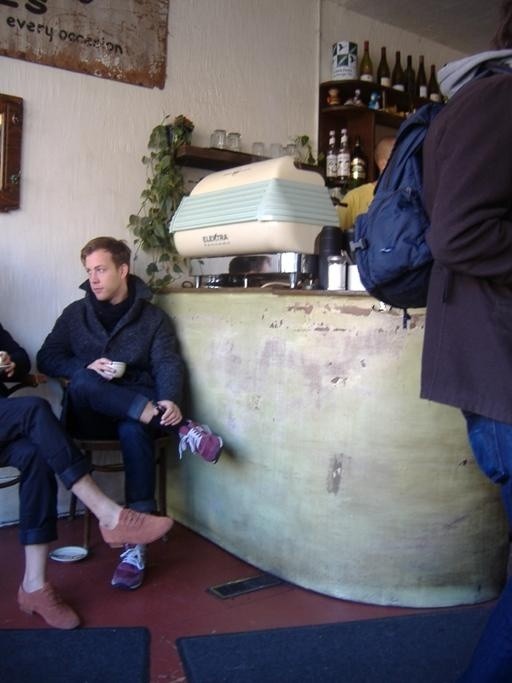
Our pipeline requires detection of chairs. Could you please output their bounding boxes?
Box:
[1,373,39,488]
[57,372,171,551]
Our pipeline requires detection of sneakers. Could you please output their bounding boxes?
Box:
[175,420,224,464]
[109,542,149,590]
[18,583,80,629]
[99,509,174,548]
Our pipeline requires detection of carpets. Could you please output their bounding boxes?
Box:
[175,609,494,683]
[0,626,151,683]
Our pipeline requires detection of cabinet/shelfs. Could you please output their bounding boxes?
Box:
[319,79,438,189]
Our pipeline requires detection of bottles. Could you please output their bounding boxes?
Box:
[404,54,416,90]
[393,50,406,92]
[351,135,368,180]
[325,130,338,182]
[377,46,390,86]
[416,54,429,99]
[360,40,374,82]
[429,64,441,103]
[338,128,351,181]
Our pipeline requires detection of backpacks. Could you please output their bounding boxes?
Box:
[350,70,489,308]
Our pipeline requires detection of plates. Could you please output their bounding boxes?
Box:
[50,544,89,562]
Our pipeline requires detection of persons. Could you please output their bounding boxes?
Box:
[335,134,396,230]
[36,236,223,593]
[416,0,511,683]
[0,323,174,631]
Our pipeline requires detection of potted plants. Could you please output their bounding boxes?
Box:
[128,114,194,290]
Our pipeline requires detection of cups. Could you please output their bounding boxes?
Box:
[210,129,226,149]
[252,142,265,156]
[228,133,242,152]
[271,144,283,159]
[104,360,128,378]
[286,143,297,155]
